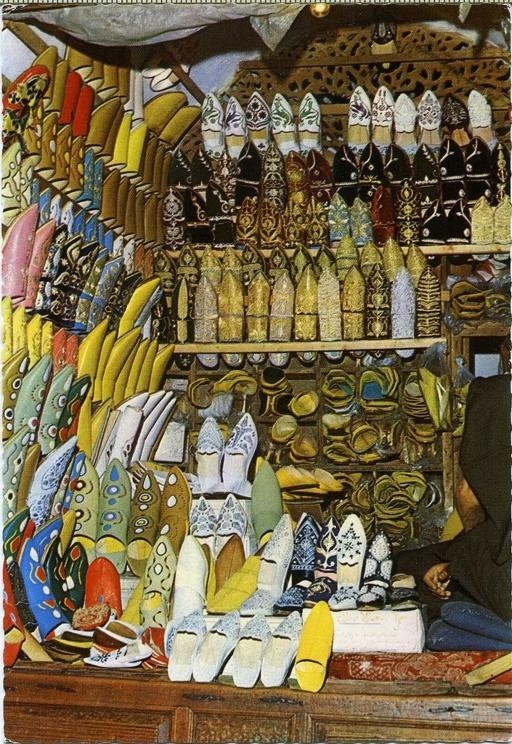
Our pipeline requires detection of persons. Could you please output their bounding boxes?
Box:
[391,374,511,622]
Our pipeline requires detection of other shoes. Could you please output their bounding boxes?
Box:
[0,38,512,340]
[3,340,419,690]
